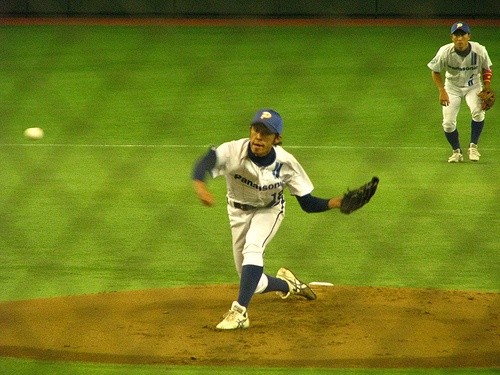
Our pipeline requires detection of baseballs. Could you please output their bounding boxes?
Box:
[22,127,43,141]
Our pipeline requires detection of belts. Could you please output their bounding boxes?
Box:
[225,198,257,211]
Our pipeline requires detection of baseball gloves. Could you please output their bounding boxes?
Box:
[339,176,379,214]
[478,88,496,111]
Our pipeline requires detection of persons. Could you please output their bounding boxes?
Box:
[427,21,496,163]
[194,110,378,332]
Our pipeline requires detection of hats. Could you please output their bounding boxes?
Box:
[250,108,282,138]
[451,22,470,34]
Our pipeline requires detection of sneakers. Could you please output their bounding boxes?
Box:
[468,143,481,161]
[448,149,463,162]
[275,266,317,299]
[215,300,251,329]
